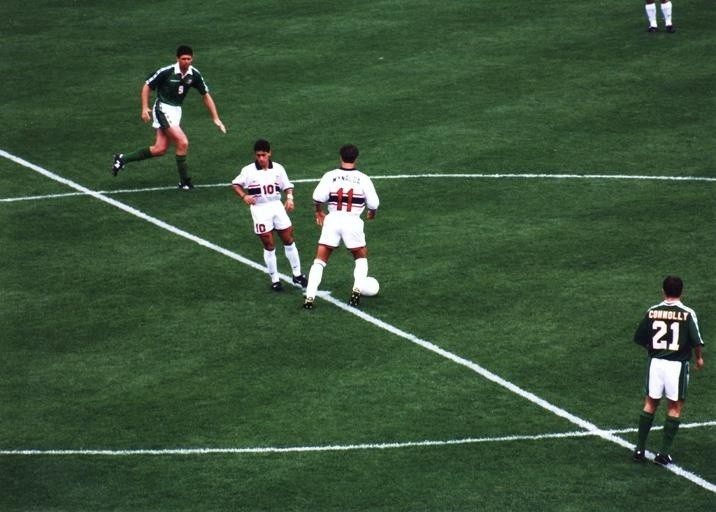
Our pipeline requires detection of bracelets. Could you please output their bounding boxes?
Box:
[241,193,248,200]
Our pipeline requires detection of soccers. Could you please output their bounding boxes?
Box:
[361,276,379,296]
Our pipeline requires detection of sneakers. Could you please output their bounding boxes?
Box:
[655,452,672,465]
[304,297,313,309]
[349,292,361,306]
[179,178,200,192]
[293,274,307,287]
[633,449,645,461]
[272,282,281,289]
[111,153,124,175]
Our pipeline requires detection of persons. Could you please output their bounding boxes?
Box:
[110,44,226,193]
[300,143,381,311]
[643,0,682,32]
[228,138,309,293]
[629,274,705,468]
